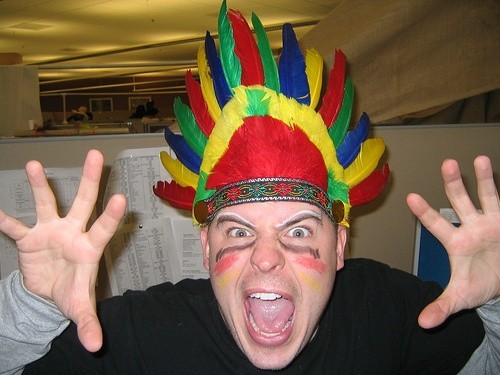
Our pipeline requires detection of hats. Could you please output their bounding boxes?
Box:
[150,0,391,228]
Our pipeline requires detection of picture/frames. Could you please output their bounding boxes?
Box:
[89,98,114,113]
[128,96,153,111]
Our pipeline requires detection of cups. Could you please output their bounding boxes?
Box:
[29,120,34,130]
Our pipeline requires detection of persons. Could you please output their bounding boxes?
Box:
[0,84,500,374]
[130,102,157,118]
[66,106,92,123]
